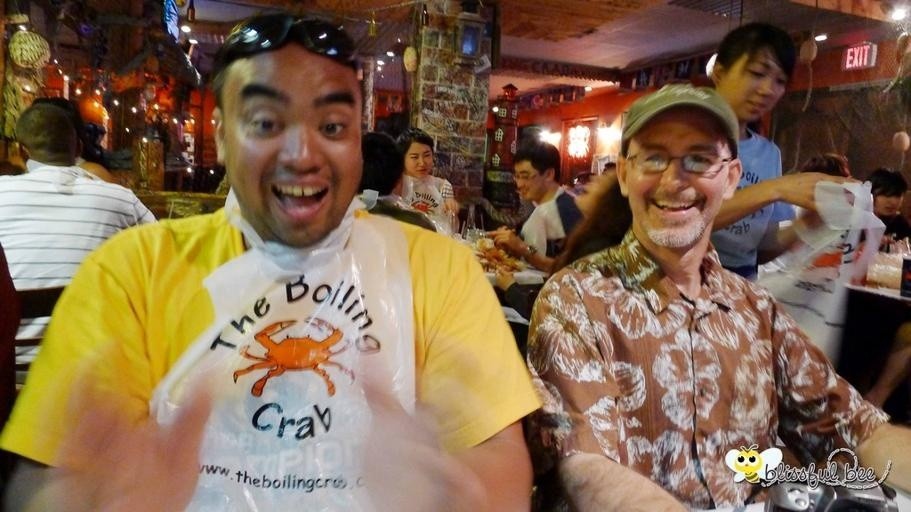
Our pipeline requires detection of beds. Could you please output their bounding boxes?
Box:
[487,240,543,291]
[845,279,910,425]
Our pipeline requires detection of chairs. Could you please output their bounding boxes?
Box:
[0,248,67,436]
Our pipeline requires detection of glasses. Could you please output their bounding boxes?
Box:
[215,10,359,67]
[622,147,734,176]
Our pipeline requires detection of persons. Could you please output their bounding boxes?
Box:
[0,1,911,512]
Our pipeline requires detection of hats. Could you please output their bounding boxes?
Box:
[620,81,742,150]
